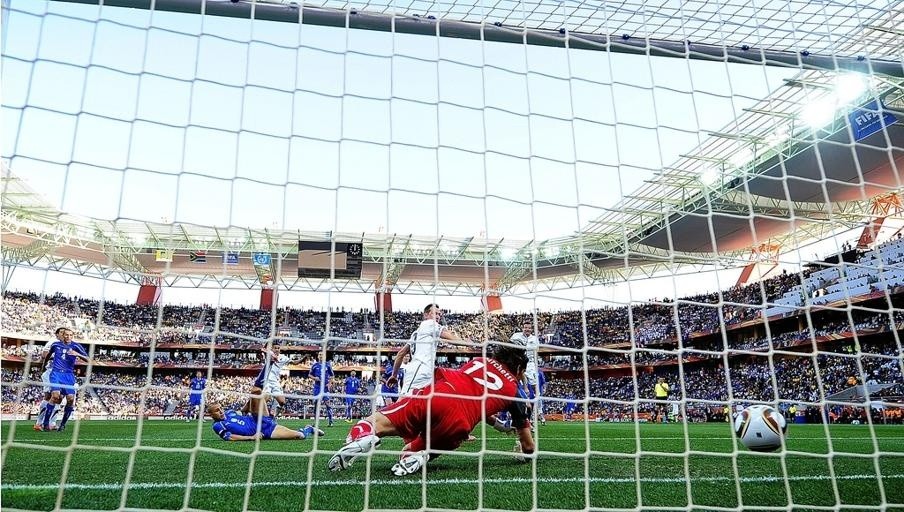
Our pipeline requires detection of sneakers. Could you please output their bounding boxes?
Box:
[259,347,280,364]
[531,429,534,434]
[327,435,381,472]
[468,435,476,441]
[305,425,324,436]
[391,450,430,477]
[33,424,65,433]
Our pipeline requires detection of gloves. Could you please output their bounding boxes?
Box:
[493,417,517,437]
[512,439,533,464]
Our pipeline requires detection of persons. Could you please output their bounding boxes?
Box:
[327,344,535,477]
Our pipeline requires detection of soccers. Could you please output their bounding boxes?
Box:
[734,405,787,452]
